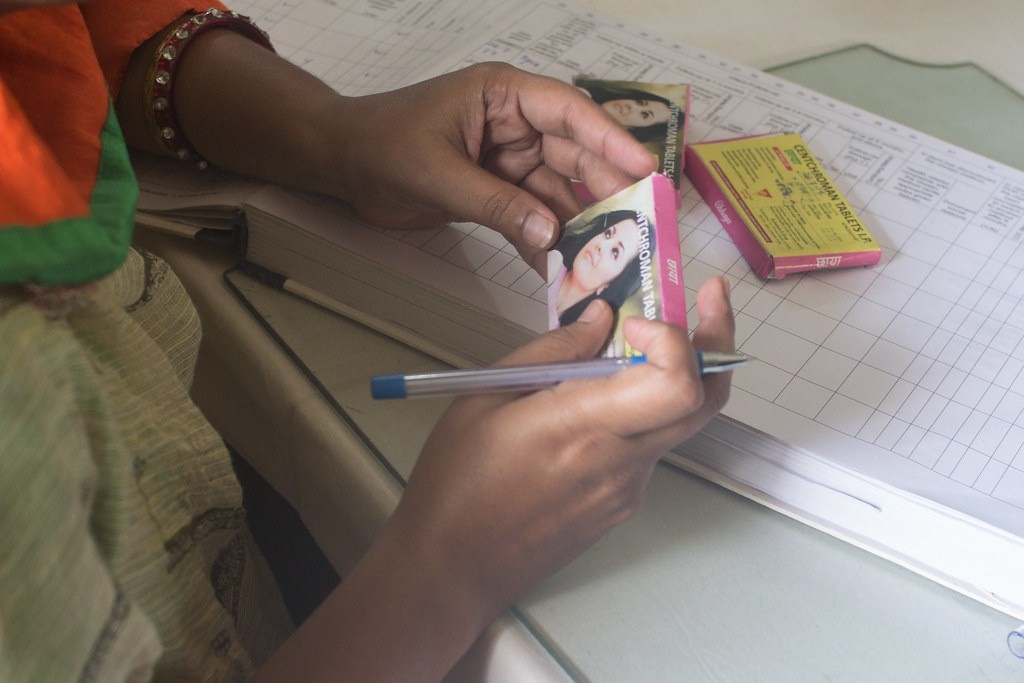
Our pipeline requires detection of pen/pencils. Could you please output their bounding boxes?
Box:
[371,349,759,401]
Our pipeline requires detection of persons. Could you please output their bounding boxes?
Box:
[547,209,656,332]
[0,0,736,683]
[579,79,684,141]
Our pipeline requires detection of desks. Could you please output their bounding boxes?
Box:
[143,0,1024,683]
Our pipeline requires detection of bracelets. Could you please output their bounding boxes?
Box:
[146,8,279,183]
